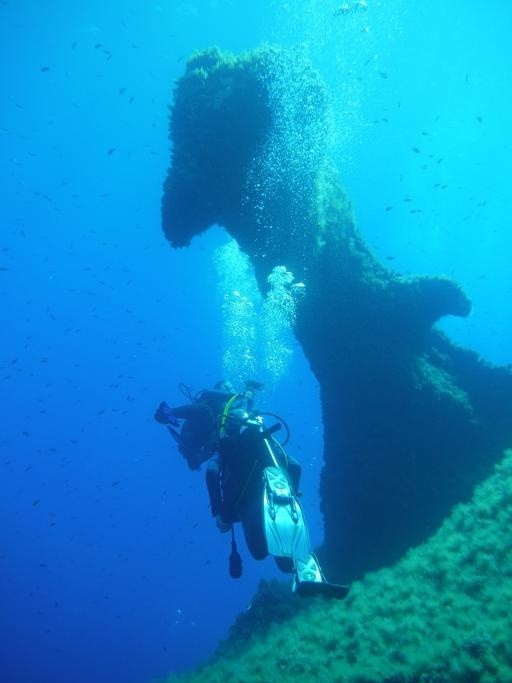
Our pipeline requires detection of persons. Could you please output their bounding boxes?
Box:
[209,381,350,600]
